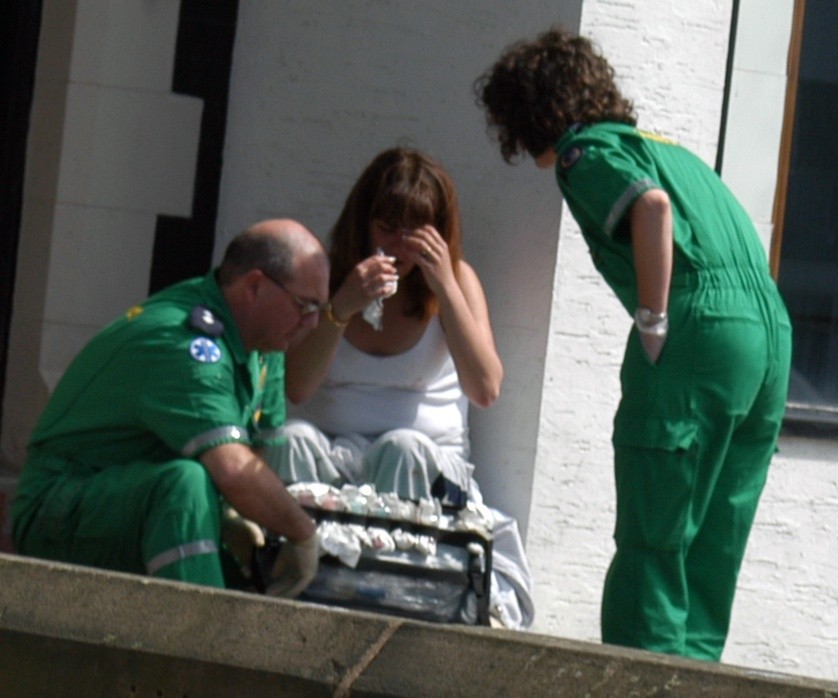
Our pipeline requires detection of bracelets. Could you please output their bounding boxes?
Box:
[634,306,667,325]
[636,323,666,337]
[322,303,357,329]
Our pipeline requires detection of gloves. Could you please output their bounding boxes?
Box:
[222,507,265,577]
[265,531,319,599]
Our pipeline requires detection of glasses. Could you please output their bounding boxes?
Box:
[263,272,332,316]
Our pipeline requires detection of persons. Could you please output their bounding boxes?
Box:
[470,24,793,661]
[11,217,330,598]
[254,143,503,512]
